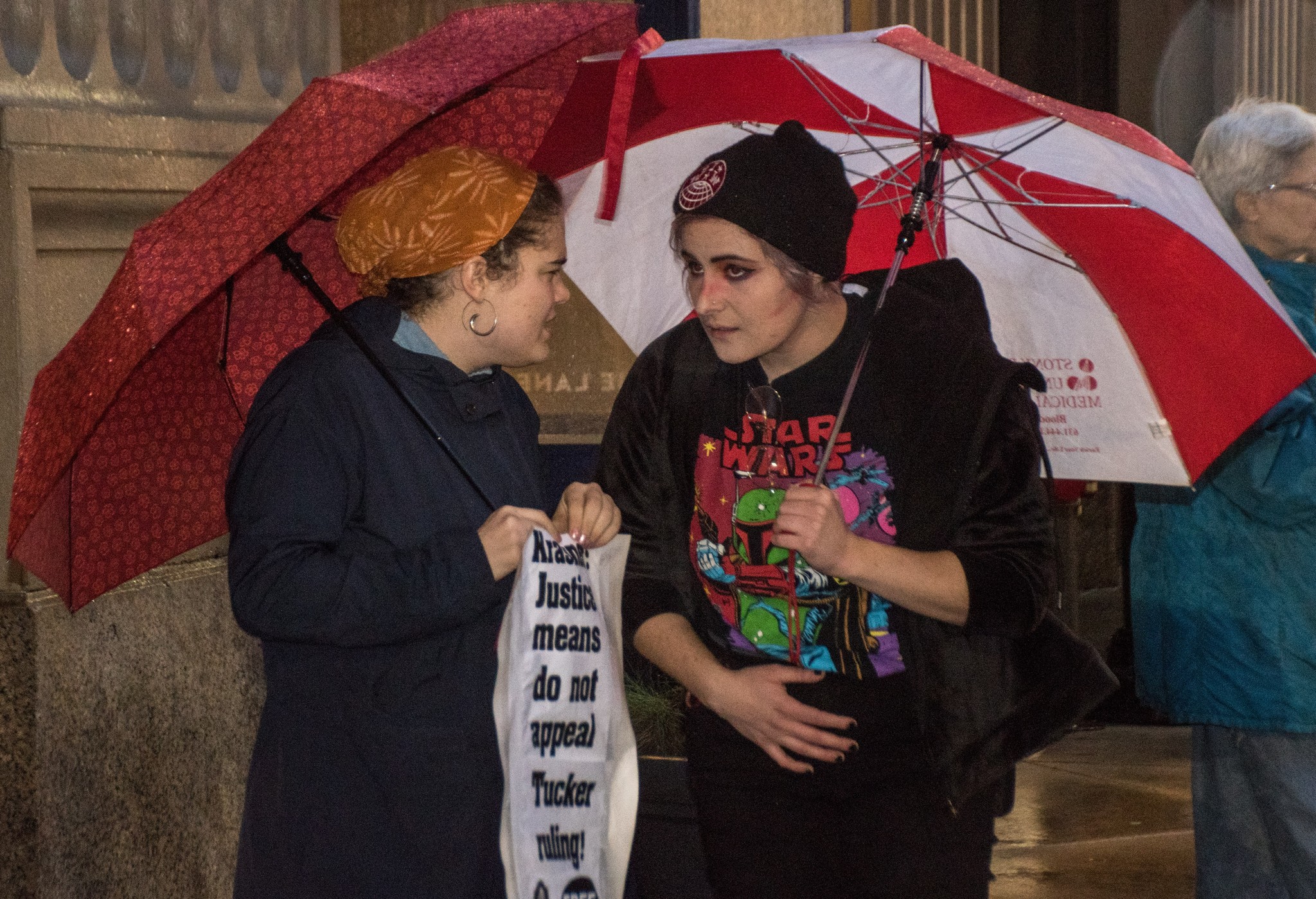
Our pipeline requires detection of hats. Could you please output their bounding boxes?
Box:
[337,145,537,281]
[672,120,859,279]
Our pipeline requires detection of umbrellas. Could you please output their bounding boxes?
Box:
[529,24,1316,493]
[6,0,639,615]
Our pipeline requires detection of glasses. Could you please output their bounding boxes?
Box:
[1253,180,1315,200]
[746,383,787,495]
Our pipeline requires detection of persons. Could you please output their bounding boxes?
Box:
[598,120,1056,899]
[1128,97,1316,899]
[227,143,622,899]
